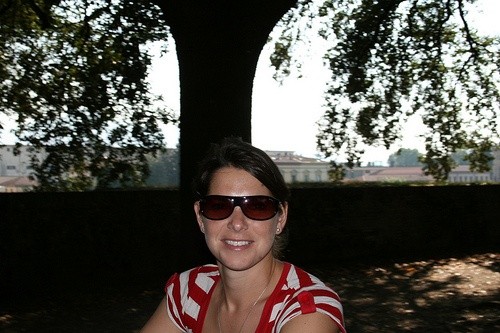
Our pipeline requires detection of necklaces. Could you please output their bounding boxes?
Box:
[218,259,277,333]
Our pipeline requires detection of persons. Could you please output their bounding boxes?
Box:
[136,140,348,333]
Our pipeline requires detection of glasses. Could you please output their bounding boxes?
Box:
[197,194,283,222]
